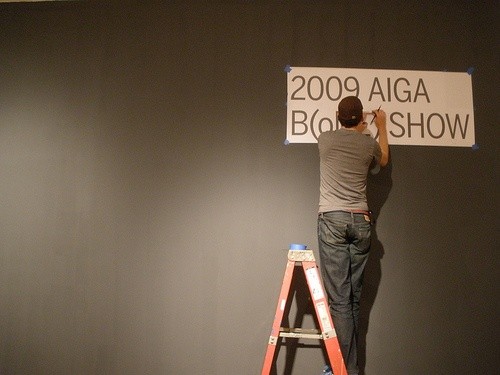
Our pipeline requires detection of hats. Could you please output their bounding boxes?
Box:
[338,95,363,121]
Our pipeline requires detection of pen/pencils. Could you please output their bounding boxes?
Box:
[370,105,382,124]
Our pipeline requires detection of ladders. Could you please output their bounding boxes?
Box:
[262,249,348,375]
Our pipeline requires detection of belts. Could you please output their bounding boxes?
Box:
[338,207,370,215]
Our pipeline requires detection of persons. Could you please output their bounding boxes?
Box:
[317,95,389,375]
